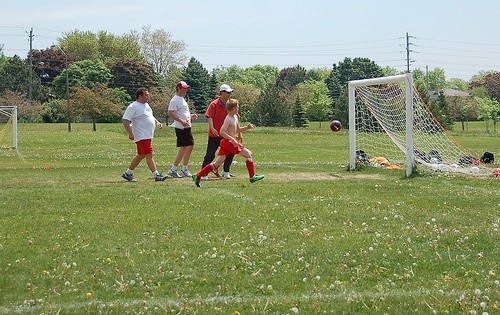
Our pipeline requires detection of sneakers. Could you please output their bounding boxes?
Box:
[200,176,208,180]
[167,168,183,178]
[250,175,265,183]
[180,169,192,178]
[154,174,166,181]
[191,172,200,187]
[121,171,137,182]
[223,171,230,179]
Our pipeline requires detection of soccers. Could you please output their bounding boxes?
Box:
[330,121,342,132]
[451,164,459,172]
[430,157,438,164]
[233,161,238,165]
[470,166,479,174]
[438,163,444,170]
[492,168,500,177]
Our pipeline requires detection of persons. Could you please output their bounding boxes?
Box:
[167,81,199,178]
[191,98,265,188]
[200,84,240,180]
[121,86,167,182]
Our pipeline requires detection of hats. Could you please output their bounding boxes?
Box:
[176,80,190,89]
[219,84,234,93]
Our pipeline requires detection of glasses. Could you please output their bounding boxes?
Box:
[226,92,231,94]
